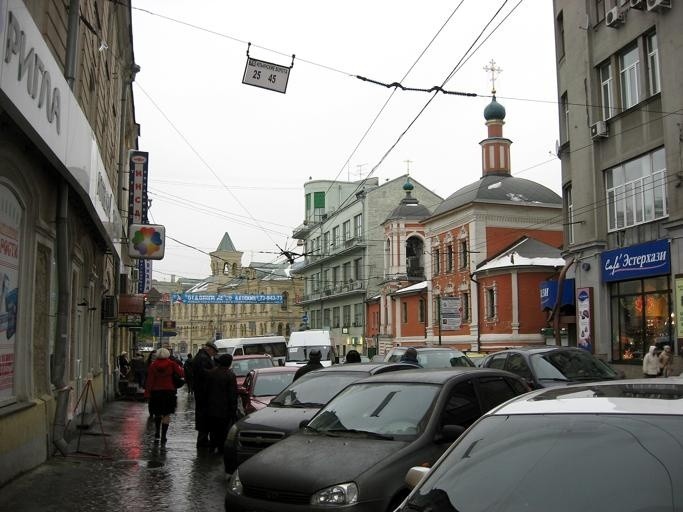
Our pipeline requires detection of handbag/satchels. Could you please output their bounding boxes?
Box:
[172,372,184,388]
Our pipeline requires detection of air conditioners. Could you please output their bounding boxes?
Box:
[589,121,607,140]
[647,0,671,12]
[101,295,117,320]
[629,0,642,8]
[352,280,362,289]
[605,7,624,28]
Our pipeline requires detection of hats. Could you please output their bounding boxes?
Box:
[346,350,361,364]
[203,341,219,353]
[155,348,170,359]
[309,348,322,361]
[404,347,418,361]
[213,354,233,366]
[120,350,127,356]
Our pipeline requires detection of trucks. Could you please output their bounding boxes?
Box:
[284,330,336,368]
[210,336,287,366]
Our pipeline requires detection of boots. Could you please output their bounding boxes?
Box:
[153,419,161,439]
[160,423,168,444]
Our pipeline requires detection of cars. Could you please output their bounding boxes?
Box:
[391,377,682,512]
[241,363,421,474]
[478,347,625,392]
[383,345,476,369]
[222,369,529,512]
[226,355,275,388]
[236,367,301,416]
[464,351,487,368]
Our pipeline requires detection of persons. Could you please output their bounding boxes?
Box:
[660,345,673,377]
[119,349,157,390]
[194,354,238,456]
[143,348,185,445]
[183,353,195,396]
[343,350,362,363]
[643,345,663,378]
[399,347,421,366]
[191,341,218,387]
[292,348,325,383]
